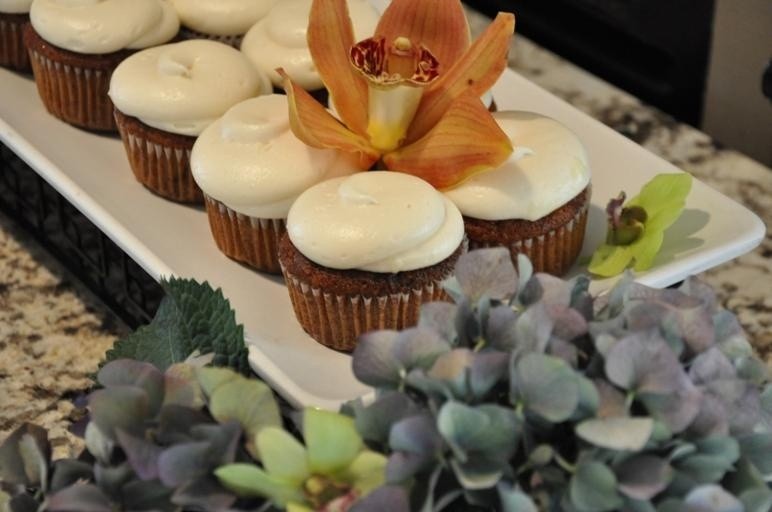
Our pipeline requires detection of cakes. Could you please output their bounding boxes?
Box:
[277,171,469,346]
[190,93,363,272]
[108,39,273,204]
[23,0,180,131]
[240,0,385,104]
[170,0,273,38]
[0,0,32,71]
[443,110,592,277]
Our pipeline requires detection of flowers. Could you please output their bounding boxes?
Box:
[1,248,772,512]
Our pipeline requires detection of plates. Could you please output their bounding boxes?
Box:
[0,0,772,416]
